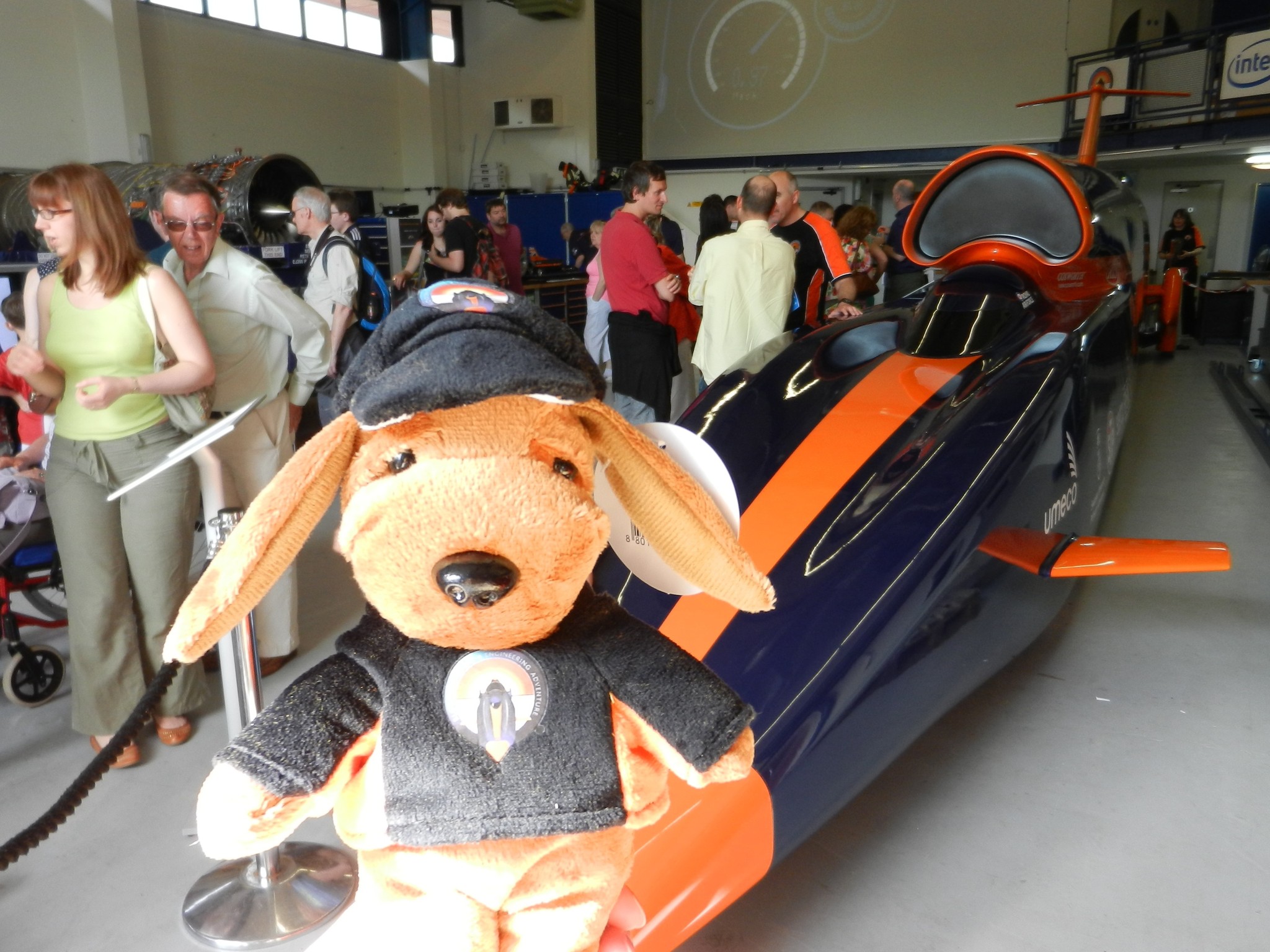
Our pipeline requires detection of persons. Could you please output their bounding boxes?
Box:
[392,204,447,289]
[601,160,682,425]
[0,162,215,770]
[472,199,522,295]
[767,171,863,333]
[290,186,364,427]
[153,173,331,679]
[427,187,479,283]
[144,183,174,269]
[1157,209,1207,326]
[688,175,796,394]
[560,179,929,316]
[328,189,366,258]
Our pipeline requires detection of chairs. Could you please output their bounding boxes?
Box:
[895,261,1039,358]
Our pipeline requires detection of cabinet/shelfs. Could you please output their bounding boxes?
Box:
[519,274,588,333]
[353,216,422,282]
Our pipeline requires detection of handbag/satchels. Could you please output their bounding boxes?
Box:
[154,342,215,431]
[853,272,880,300]
[0,464,51,524]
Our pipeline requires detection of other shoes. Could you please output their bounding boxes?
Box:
[89,735,138,767]
[261,649,300,678]
[154,715,193,747]
[201,650,221,672]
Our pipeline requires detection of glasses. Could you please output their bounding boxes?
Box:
[31,208,71,221]
[165,214,218,232]
[290,209,304,218]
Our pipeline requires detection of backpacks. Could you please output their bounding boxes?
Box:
[459,215,509,289]
[323,241,392,329]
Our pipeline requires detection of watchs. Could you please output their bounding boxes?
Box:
[839,298,853,305]
[675,274,680,279]
[130,376,140,395]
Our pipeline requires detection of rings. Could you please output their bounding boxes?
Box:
[39,472,44,477]
[393,278,397,281]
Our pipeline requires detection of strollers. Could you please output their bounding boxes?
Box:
[0,466,70,707]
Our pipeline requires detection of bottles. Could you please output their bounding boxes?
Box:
[367,291,380,324]
[875,228,885,241]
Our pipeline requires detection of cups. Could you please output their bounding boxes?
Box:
[529,172,547,194]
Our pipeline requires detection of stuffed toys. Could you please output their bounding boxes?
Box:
[162,277,777,952]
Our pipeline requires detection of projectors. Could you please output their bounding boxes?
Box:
[383,204,418,217]
